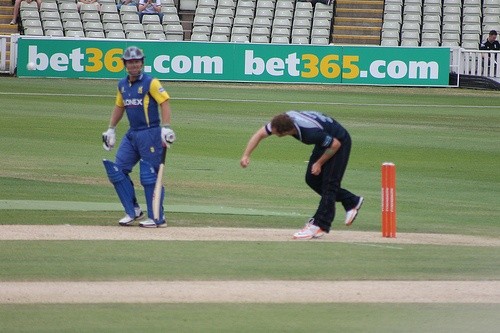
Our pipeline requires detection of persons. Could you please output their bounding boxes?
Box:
[76,0,101,14]
[99,47,176,228]
[10,0,42,25]
[480,30,500,70]
[240,111,364,240]
[138,0,164,25]
[116,0,139,14]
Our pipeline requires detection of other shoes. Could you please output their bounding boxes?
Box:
[10,20,17,25]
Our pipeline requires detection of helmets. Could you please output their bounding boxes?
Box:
[121,47,146,76]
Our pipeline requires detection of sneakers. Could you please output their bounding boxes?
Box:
[117,206,146,225]
[139,218,167,228]
[294,218,329,239]
[344,196,365,225]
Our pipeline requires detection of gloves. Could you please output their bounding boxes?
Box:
[160,127,175,148]
[102,128,115,150]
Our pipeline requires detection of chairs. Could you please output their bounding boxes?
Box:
[191,0,334,44]
[381,0,500,50]
[19,0,184,40]
